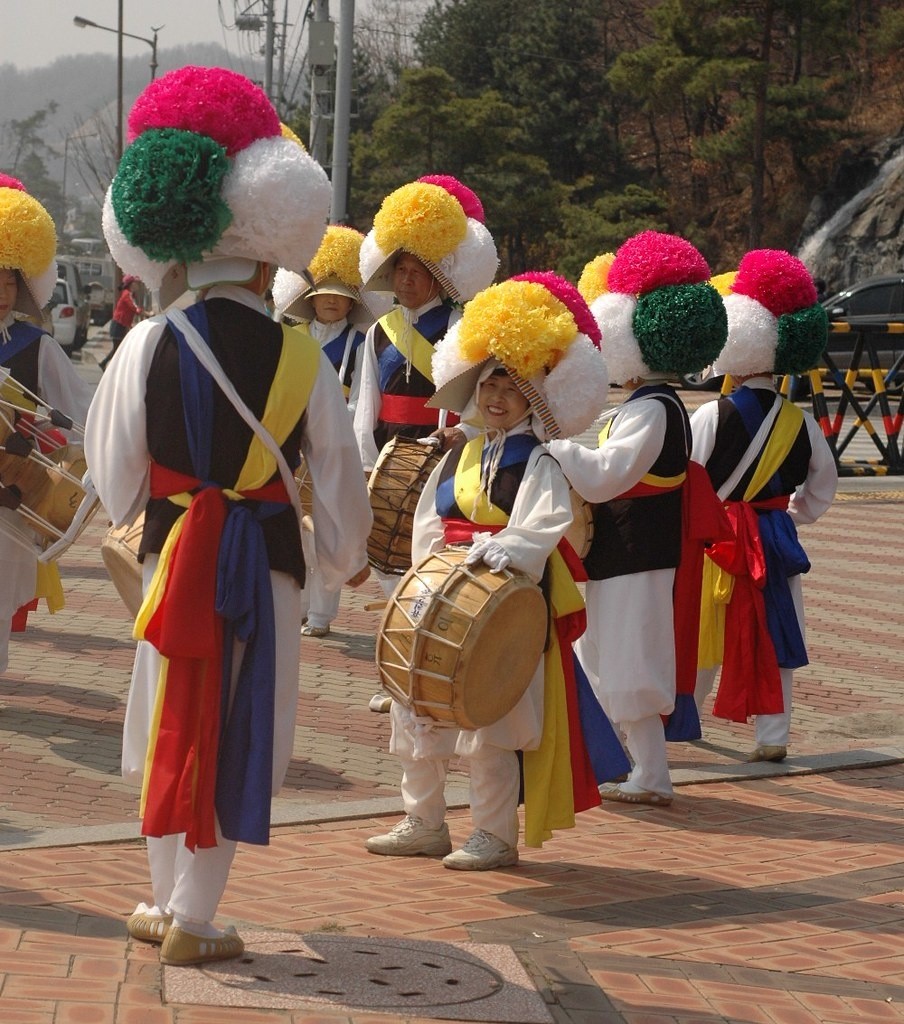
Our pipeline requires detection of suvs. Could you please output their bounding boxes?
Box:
[49,238,121,359]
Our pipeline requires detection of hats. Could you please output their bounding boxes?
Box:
[122,274,140,287]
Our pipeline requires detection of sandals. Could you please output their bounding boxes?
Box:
[158,925,246,966]
[745,745,789,763]
[299,624,333,637]
[596,781,673,807]
[125,910,174,945]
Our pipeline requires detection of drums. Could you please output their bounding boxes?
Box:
[375,545,548,732]
[0,368,103,563]
[553,470,602,569]
[362,438,448,577]
[101,509,146,621]
[291,448,313,521]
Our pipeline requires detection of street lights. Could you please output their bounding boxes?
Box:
[72,15,166,82]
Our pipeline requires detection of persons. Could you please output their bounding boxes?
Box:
[82,65,369,964]
[541,228,727,808]
[268,222,397,633]
[352,173,500,599]
[361,271,631,869]
[97,274,155,371]
[685,247,836,765]
[1,170,95,676]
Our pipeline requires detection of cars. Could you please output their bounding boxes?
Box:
[816,273,904,395]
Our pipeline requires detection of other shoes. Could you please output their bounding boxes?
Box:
[96,362,108,372]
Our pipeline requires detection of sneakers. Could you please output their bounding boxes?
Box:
[440,827,519,871]
[362,814,454,859]
